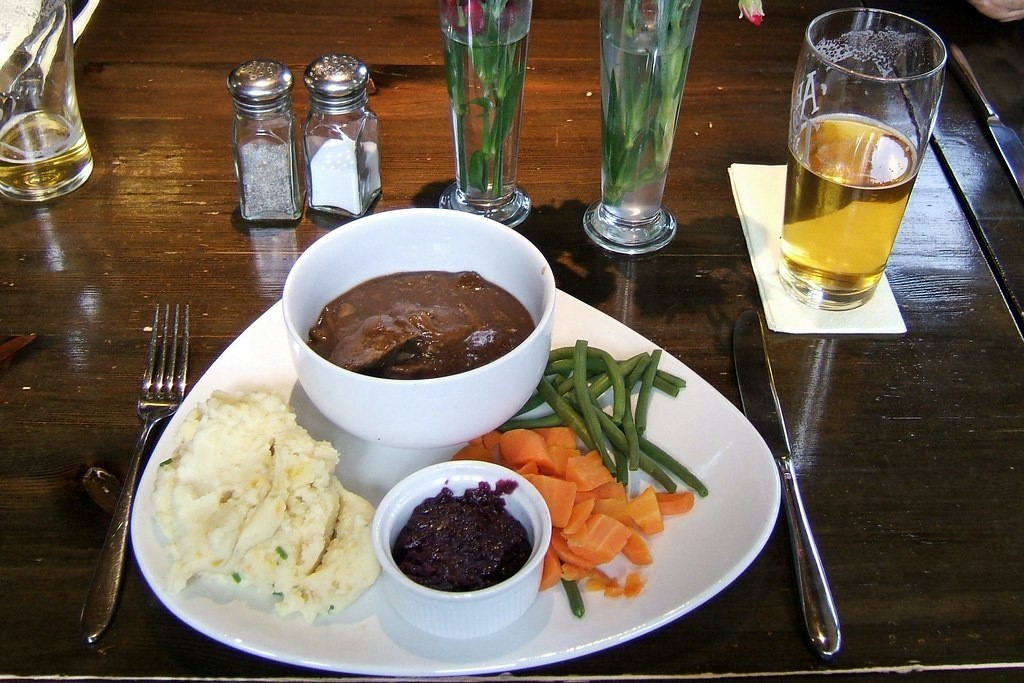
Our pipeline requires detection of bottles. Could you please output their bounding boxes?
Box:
[227,56,305,223]
[302,54,384,219]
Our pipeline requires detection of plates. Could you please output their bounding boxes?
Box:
[129,285,784,677]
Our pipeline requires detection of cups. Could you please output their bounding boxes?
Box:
[583,0,704,255]
[437,0,533,230]
[0,0,94,204]
[777,6,948,311]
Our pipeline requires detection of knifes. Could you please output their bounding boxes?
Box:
[949,44,1024,199]
[731,307,845,658]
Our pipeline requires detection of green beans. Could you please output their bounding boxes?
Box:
[497,340,708,499]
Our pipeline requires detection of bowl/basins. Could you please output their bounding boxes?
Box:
[374,459,551,641]
[282,207,557,454]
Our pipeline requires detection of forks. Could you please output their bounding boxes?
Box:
[80,299,192,648]
